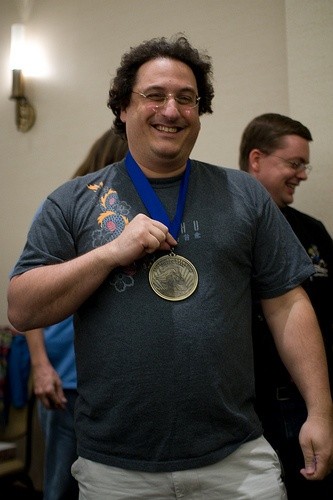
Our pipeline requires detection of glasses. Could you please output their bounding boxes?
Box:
[132,91,201,109]
[267,155,312,174]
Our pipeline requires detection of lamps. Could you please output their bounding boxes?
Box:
[8,23,34,133]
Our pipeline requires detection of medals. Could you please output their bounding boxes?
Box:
[149,254,199,301]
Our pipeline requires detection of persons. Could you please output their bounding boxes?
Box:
[240,113,333,500]
[7,36,332,500]
[23,128,129,500]
